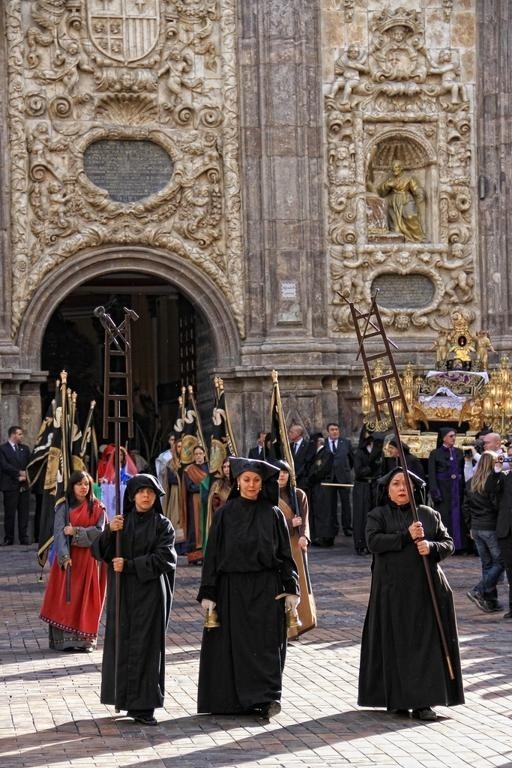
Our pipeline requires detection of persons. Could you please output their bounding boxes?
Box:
[352,422,384,558]
[388,436,425,479]
[163,439,185,556]
[310,432,335,480]
[377,158,428,245]
[469,433,507,482]
[357,467,466,722]
[324,421,355,538]
[483,441,512,622]
[427,427,464,555]
[96,445,115,479]
[196,456,300,721]
[248,432,266,460]
[469,453,503,615]
[287,423,312,491]
[91,472,177,726]
[39,468,109,653]
[102,447,133,517]
[205,455,235,543]
[0,424,33,548]
[270,459,318,647]
[155,431,174,486]
[264,432,272,453]
[181,446,208,565]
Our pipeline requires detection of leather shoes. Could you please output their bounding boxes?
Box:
[20,538,32,545]
[504,613,512,619]
[261,700,281,720]
[387,707,409,717]
[343,530,352,537]
[412,706,437,720]
[134,715,158,727]
[0,540,13,546]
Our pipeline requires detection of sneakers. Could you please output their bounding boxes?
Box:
[466,589,488,613]
[494,604,504,611]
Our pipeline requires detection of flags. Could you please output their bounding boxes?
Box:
[206,376,239,476]
[25,368,82,569]
[82,401,97,471]
[268,367,298,488]
[172,386,205,471]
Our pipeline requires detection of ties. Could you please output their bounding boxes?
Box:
[14,445,18,451]
[259,448,263,455]
[293,443,297,453]
[332,440,337,455]
